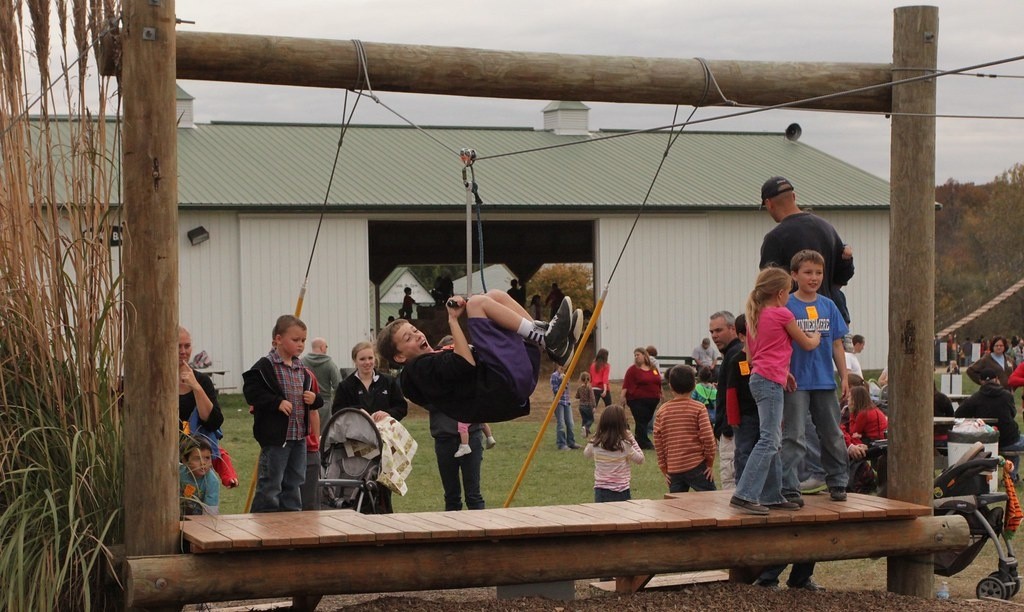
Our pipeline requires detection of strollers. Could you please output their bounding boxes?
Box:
[318,409,386,513]
[934,440,1022,600]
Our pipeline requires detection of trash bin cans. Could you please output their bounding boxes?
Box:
[947,430,999,492]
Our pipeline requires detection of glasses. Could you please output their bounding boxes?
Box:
[994,344,1004,348]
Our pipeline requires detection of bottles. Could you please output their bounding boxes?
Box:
[936,580,950,601]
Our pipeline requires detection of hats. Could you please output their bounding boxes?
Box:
[760,176,794,211]
[980,367,998,382]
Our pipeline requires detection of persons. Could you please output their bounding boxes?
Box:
[243,315,325,513]
[550,367,585,451]
[759,176,855,493]
[575,371,596,438]
[836,334,1024,495]
[653,364,716,494]
[429,335,485,512]
[178,327,239,516]
[301,338,343,436]
[729,267,821,514]
[590,309,824,591]
[249,326,321,510]
[583,404,645,503]
[506,279,593,321]
[385,287,420,325]
[377,289,583,424]
[782,249,849,506]
[434,335,496,457]
[331,341,408,422]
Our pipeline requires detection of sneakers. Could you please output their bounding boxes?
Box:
[486,436,496,450]
[543,295,572,357]
[548,308,584,368]
[454,444,472,458]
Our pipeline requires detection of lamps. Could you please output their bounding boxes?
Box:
[187,226,209,245]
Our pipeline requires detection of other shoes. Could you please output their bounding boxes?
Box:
[569,443,582,450]
[729,495,770,515]
[798,475,828,493]
[782,493,804,507]
[581,426,588,438]
[558,445,571,451]
[639,438,654,449]
[829,485,847,501]
[762,501,800,511]
